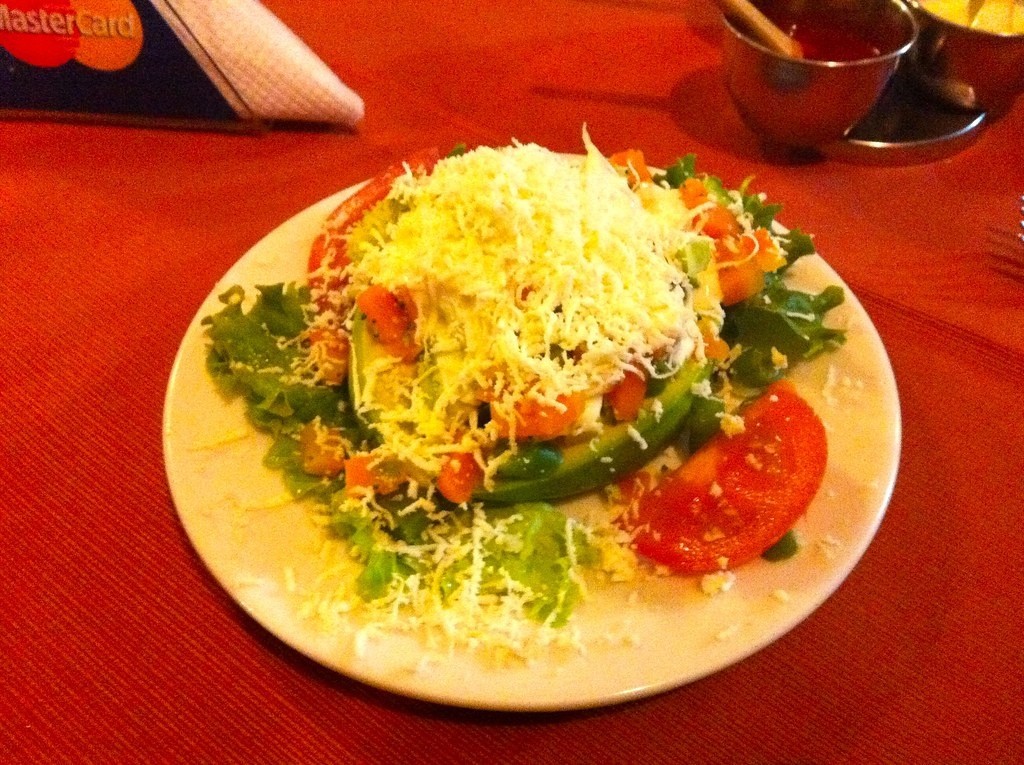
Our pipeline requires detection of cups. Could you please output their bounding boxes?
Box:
[713,0,918,147]
[910,0,1024,122]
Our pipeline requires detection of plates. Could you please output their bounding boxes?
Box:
[162,152,903,712]
[843,69,986,148]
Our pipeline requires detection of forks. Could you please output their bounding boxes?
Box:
[1016,195,1024,243]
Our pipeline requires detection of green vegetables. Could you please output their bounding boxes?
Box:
[196,151,846,542]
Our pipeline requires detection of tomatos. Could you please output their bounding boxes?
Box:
[308,147,443,385]
[615,383,829,579]
